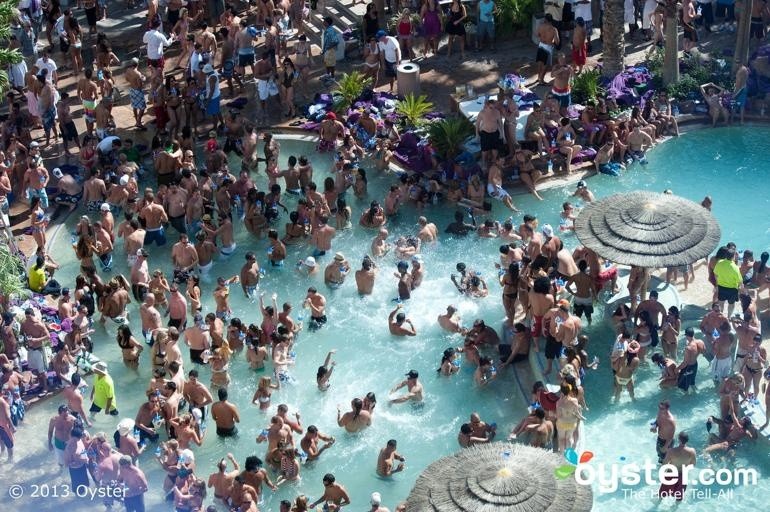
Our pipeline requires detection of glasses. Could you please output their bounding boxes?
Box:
[170,79,196,86]
[546,95,554,101]
[187,155,195,159]
[587,108,594,111]
[283,62,290,66]
[324,483,330,486]
[461,319,523,349]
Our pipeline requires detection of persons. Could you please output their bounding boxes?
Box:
[0,1,769,510]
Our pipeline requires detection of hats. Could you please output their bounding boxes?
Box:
[404,370,418,380]
[201,63,214,73]
[29,141,39,149]
[541,223,555,238]
[557,298,571,311]
[539,389,560,412]
[374,30,387,39]
[370,491,382,507]
[192,408,202,425]
[52,168,64,179]
[100,202,110,212]
[199,213,230,224]
[305,256,317,267]
[218,388,228,401]
[119,174,129,186]
[90,361,108,376]
[325,111,337,120]
[321,16,333,26]
[116,417,136,436]
[130,57,140,65]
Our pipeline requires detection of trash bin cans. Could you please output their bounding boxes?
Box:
[396,63,420,100]
[531,14,548,46]
